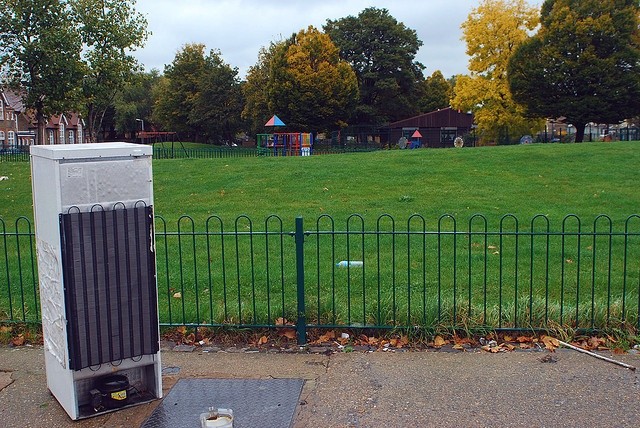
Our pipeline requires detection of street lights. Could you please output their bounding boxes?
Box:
[135,119,144,144]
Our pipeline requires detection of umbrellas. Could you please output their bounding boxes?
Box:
[264,115,286,134]
